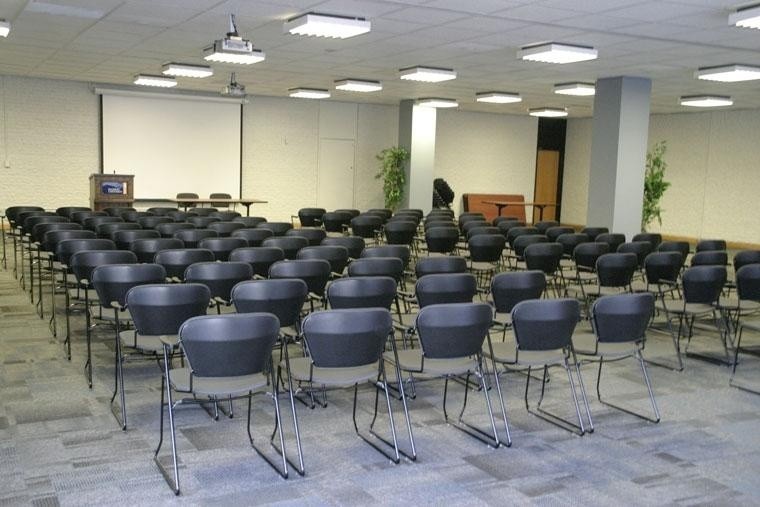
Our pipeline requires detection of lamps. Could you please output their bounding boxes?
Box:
[0,18,11,37]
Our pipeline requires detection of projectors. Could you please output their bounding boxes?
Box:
[214,31,252,55]
[221,82,246,97]
